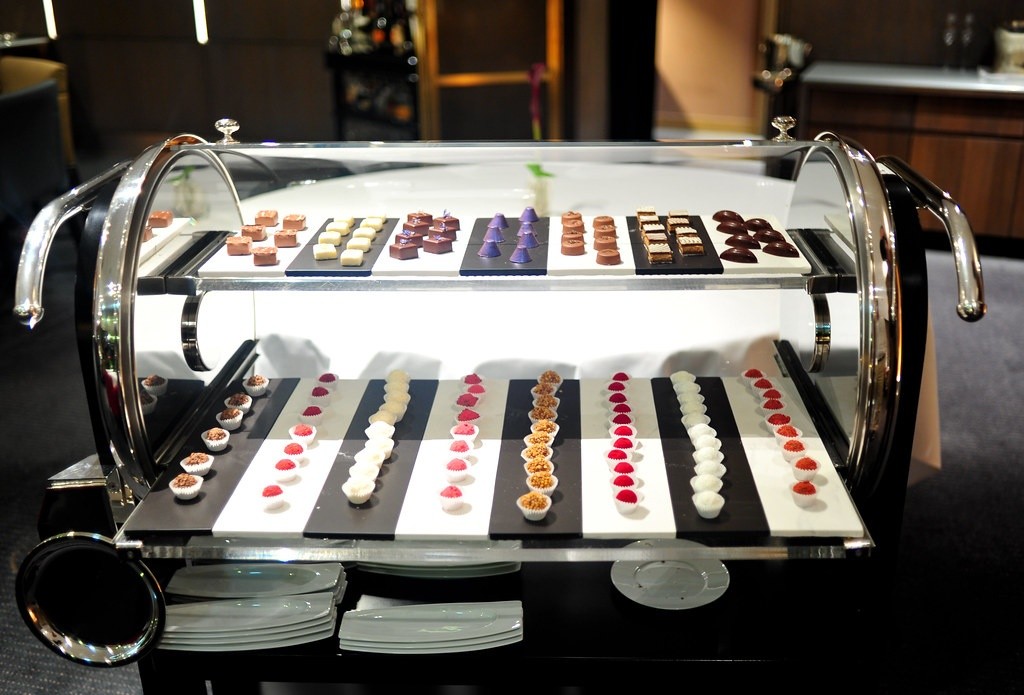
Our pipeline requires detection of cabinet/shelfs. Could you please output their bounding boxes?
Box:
[120,193,883,576]
[797,91,1021,247]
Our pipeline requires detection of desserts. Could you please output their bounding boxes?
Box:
[137,373,168,413]
[439,373,487,511]
[167,374,266,498]
[478,207,539,263]
[604,372,640,511]
[258,372,340,509]
[669,371,727,519]
[515,369,564,521]
[390,210,460,260]
[342,369,411,505]
[312,213,387,269]
[636,209,706,263]
[227,210,307,266]
[561,211,621,265]
[713,209,799,264]
[740,369,821,504]
